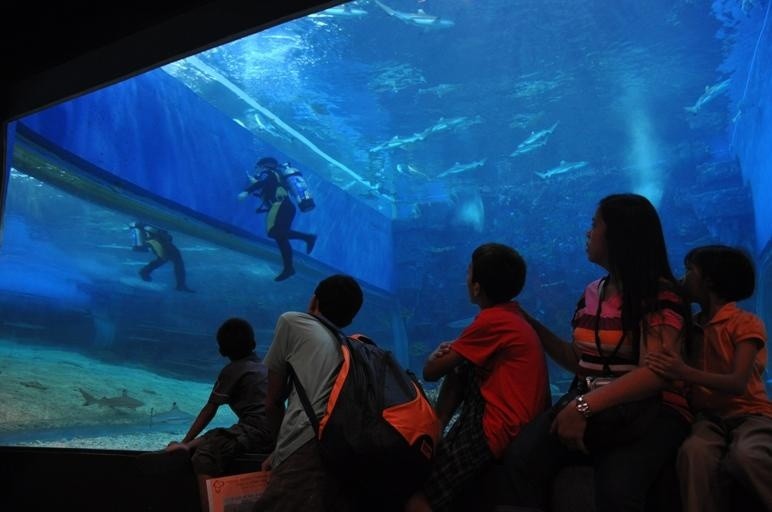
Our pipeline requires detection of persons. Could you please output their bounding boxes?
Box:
[250,271,365,509]
[160,316,271,512]
[234,153,319,282]
[402,241,554,512]
[640,237,771,512]
[503,190,690,512]
[127,220,199,294]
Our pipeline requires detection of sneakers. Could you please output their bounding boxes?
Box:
[138,268,152,281]
[176,284,195,293]
[305,234,316,254]
[275,267,294,281]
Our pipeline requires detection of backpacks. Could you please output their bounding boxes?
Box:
[286,311,444,512]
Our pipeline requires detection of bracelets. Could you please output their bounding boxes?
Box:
[574,395,594,420]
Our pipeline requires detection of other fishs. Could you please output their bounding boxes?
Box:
[396,163,432,183]
[436,158,487,176]
[377,1,456,31]
[19,379,48,391]
[367,114,465,152]
[508,119,560,155]
[680,77,731,117]
[318,3,368,17]
[144,401,196,422]
[533,160,589,179]
[76,384,148,411]
[417,81,458,96]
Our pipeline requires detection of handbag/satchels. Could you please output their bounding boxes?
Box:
[541,379,664,465]
